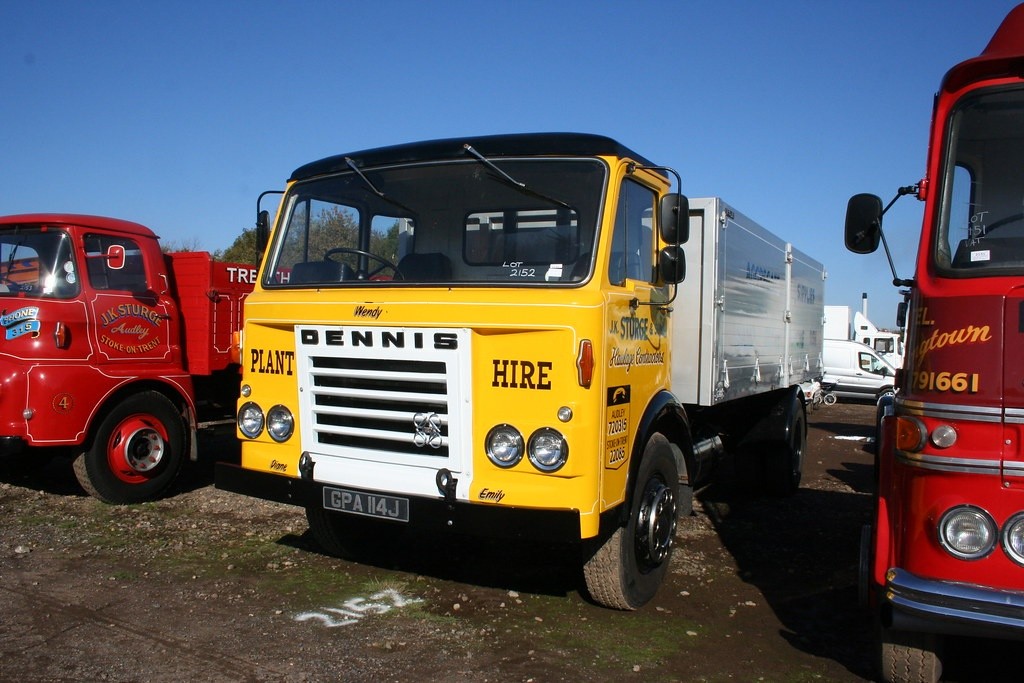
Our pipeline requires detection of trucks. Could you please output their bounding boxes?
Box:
[0,213,392,505]
[235,132,826,611]
[822,307,904,366]
[844,0,1024,683]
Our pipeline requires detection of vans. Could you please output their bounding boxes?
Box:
[800,338,902,412]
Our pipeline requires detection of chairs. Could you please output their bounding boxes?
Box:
[394,253,452,282]
[570,253,640,285]
